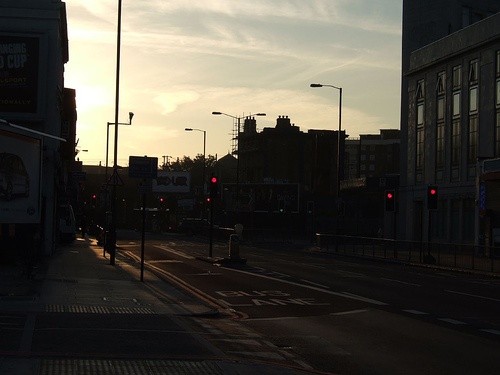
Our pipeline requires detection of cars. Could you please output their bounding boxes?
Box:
[176,217,235,240]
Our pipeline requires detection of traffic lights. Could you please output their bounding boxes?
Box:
[208,176,219,198]
[383,189,396,211]
[426,184,438,210]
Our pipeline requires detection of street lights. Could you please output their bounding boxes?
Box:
[212,111,266,217]
[186,128,207,218]
[311,82,342,245]
